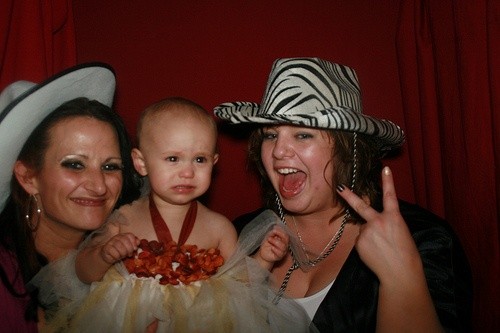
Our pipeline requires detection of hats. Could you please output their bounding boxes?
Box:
[0,66,115,213]
[213,57,407,148]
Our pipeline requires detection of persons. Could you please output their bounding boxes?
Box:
[213,54,473,333]
[0,62,143,333]
[28,98,310,333]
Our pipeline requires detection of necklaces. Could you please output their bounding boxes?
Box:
[293,214,341,267]
